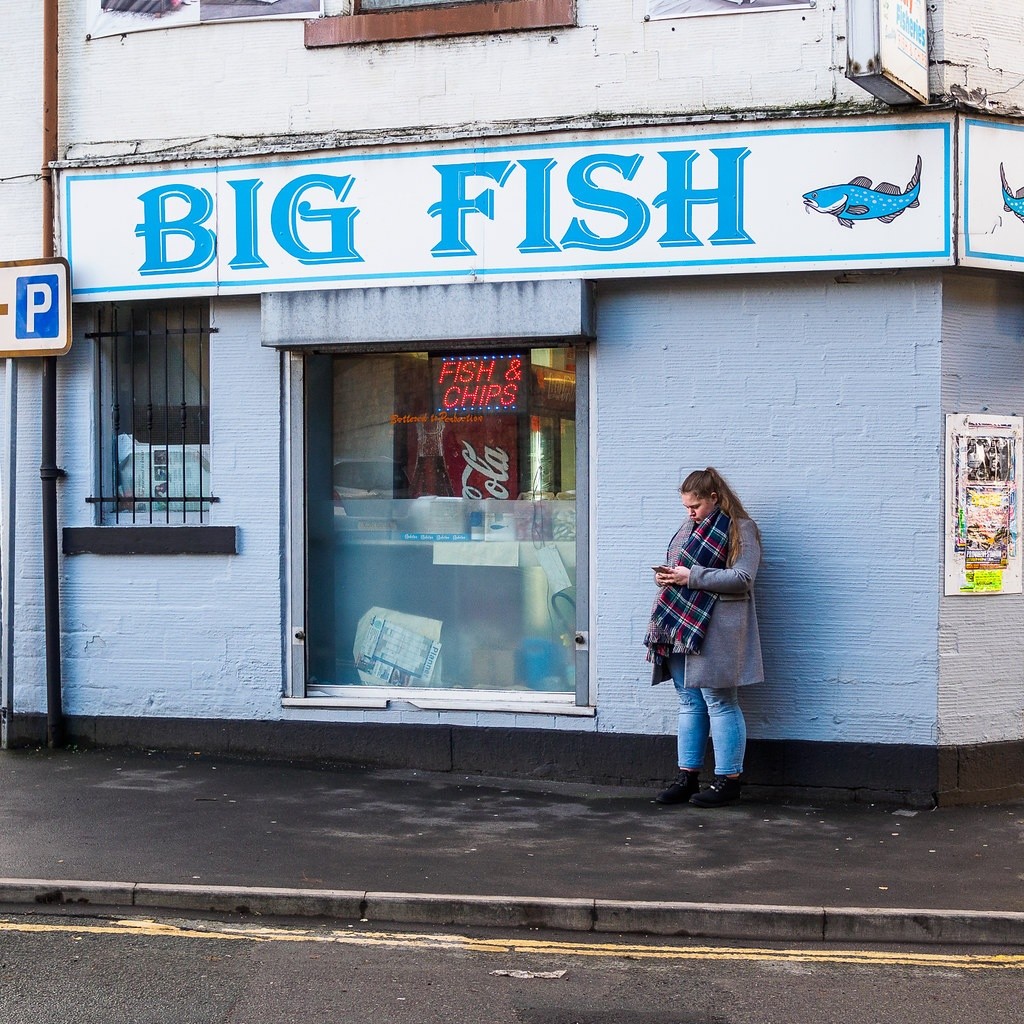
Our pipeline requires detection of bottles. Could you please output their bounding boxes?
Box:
[408,416,456,500]
[515,490,575,540]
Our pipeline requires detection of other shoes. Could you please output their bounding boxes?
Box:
[690,778,741,806]
[656,771,700,802]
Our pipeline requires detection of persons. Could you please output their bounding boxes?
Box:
[966,438,1012,482]
[643,467,764,806]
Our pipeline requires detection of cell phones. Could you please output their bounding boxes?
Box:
[651,566,668,575]
